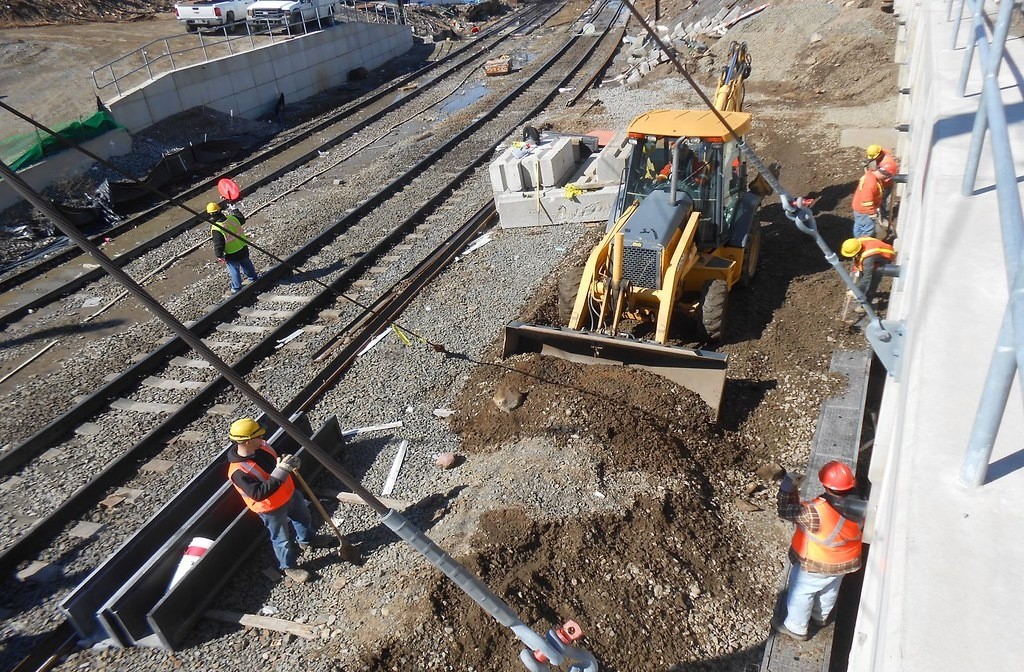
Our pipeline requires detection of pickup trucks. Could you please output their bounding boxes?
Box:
[175,0,255,33]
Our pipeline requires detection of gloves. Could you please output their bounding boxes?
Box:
[780,470,807,494]
[276,454,301,473]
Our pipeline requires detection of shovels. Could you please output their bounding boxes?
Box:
[864,166,894,235]
[281,452,369,569]
[841,276,858,321]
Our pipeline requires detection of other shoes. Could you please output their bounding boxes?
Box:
[241,279,253,286]
[855,305,866,313]
[810,615,827,626]
[846,289,856,298]
[284,563,311,582]
[769,617,808,641]
[299,543,315,552]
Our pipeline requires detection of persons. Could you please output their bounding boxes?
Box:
[851,146,900,241]
[226,418,333,582]
[656,142,708,191]
[840,236,896,312]
[206,203,257,294]
[770,462,865,640]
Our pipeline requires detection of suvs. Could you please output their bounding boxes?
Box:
[246,0,341,35]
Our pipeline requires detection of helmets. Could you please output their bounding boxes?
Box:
[206,202,221,213]
[228,418,266,441]
[818,460,857,491]
[841,238,862,257]
[867,144,882,159]
[878,163,898,177]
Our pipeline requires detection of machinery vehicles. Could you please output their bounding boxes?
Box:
[498,108,781,425]
